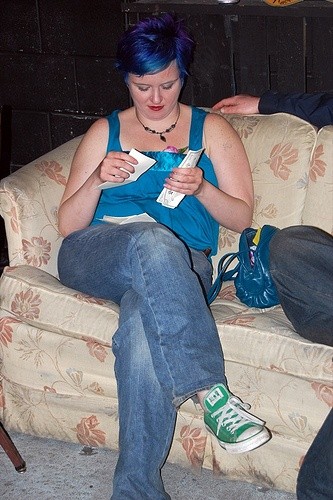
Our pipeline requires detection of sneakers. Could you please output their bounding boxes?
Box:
[203,384,270,455]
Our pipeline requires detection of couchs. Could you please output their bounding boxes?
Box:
[0,106,333,495]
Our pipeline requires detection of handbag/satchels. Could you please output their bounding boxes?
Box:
[206,225,281,308]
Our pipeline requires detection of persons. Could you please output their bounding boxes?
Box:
[58,14,272,500]
[211,90,333,500]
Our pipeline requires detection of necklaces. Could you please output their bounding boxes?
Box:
[135,104,181,143]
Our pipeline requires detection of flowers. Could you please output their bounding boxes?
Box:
[163,146,189,153]
